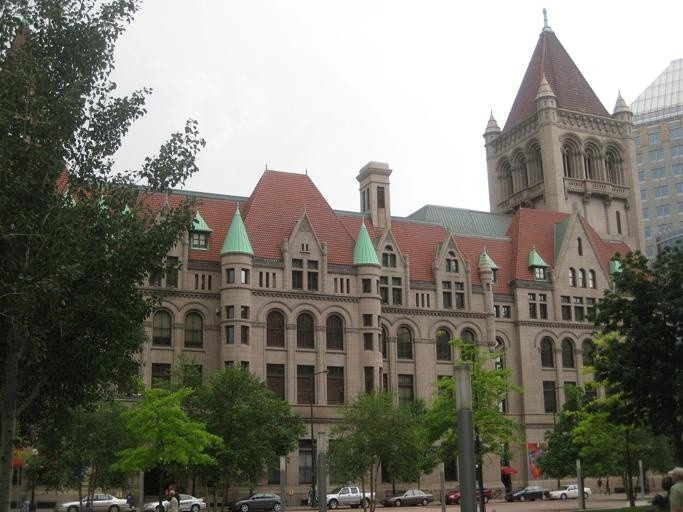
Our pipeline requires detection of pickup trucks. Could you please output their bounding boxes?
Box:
[315,486,377,509]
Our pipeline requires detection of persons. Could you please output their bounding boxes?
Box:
[170,490,178,512]
[669,467,683,512]
[598,478,602,493]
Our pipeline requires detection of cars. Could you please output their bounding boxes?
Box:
[505,485,591,502]
[440,483,492,504]
[55,494,134,512]
[381,489,434,507]
[143,493,208,512]
[228,492,287,511]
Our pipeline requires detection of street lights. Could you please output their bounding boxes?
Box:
[29,448,39,511]
[310,369,330,505]
